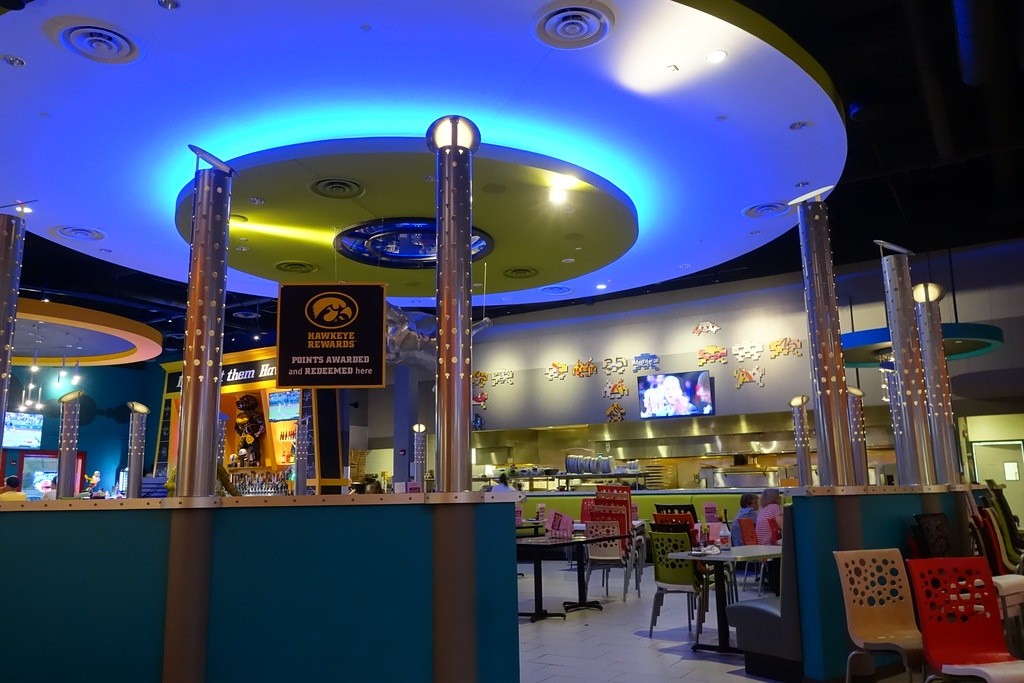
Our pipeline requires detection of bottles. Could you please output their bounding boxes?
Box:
[720,522,731,551]
[535,510,539,520]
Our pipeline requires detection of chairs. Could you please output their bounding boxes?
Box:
[564,483,783,649]
[833,479,1024,683]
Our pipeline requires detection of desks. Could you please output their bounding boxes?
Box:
[516,517,631,624]
[668,545,781,655]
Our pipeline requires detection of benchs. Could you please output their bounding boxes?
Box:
[725,503,804,682]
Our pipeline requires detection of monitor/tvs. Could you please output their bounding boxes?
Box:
[268,390,300,422]
[2,412,43,450]
[637,370,714,419]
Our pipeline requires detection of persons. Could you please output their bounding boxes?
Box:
[755,489,783,546]
[641,372,714,418]
[1,475,27,502]
[730,495,759,546]
[491,474,517,493]
[41,476,58,499]
[234,395,266,467]
